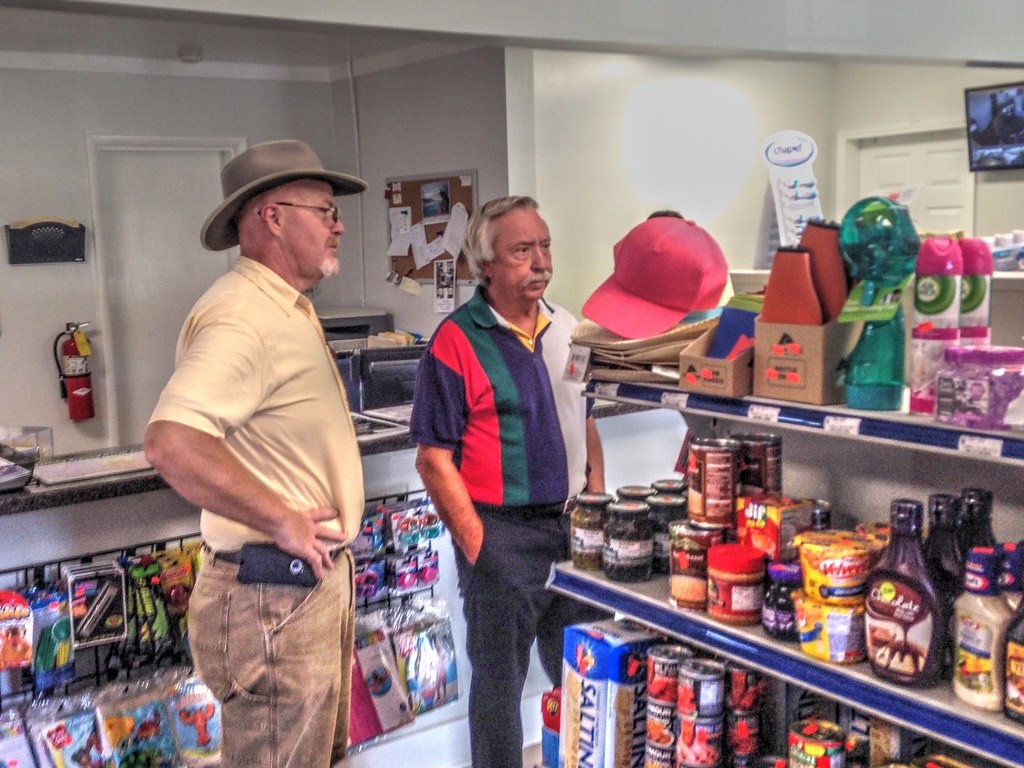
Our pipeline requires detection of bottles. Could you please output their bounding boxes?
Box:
[961,489,995,556]
[762,562,807,642]
[923,493,964,683]
[707,544,766,626]
[863,498,943,689]
[954,540,1024,723]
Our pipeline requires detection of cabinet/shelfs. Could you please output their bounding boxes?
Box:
[545,268,1024,768]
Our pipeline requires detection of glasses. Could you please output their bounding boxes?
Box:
[258,201,341,222]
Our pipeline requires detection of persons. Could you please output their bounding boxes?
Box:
[143,139,370,768]
[408,194,608,768]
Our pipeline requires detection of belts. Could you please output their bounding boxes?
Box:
[200,540,241,564]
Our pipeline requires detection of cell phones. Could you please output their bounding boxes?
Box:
[237,542,318,587]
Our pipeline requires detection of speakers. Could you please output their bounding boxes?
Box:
[965,80,1024,171]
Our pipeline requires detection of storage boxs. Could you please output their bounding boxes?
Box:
[851,714,930,764]
[678,325,755,398]
[753,313,866,406]
[558,617,665,768]
[736,491,815,564]
[542,726,559,768]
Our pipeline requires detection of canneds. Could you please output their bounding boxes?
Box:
[644,644,866,768]
[566,433,807,643]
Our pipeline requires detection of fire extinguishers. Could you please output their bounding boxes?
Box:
[53,321,95,420]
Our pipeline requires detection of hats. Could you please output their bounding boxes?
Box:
[199,140,369,251]
[568,216,729,384]
